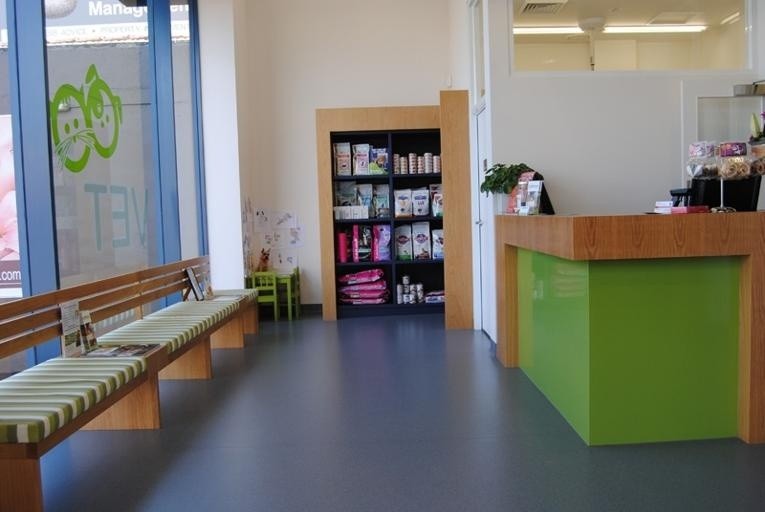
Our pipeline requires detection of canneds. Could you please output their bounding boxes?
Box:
[396,276,424,304]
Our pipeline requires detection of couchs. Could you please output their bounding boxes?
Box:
[0,254,260,511]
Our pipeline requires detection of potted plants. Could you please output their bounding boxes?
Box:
[480,163,534,214]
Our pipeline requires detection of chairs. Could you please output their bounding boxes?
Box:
[278,266,301,320]
[252,271,280,321]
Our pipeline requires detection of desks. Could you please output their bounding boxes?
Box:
[246,272,296,320]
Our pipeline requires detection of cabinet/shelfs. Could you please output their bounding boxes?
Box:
[329,128,446,320]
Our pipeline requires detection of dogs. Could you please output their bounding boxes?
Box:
[255,248,271,272]
